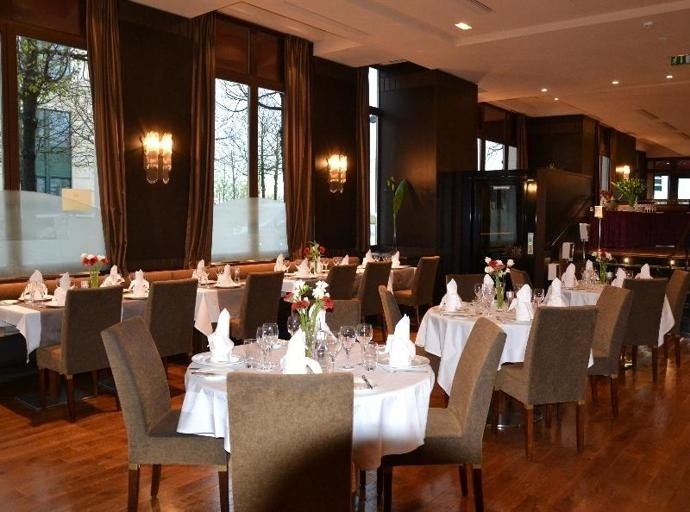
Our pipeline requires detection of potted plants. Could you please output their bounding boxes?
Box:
[383,173,410,254]
[613,177,649,212]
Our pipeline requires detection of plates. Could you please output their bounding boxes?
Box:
[19,294,53,300]
[45,302,64,308]
[214,284,240,288]
[438,310,471,316]
[123,294,149,300]
[198,280,215,285]
[381,353,432,370]
[190,351,244,367]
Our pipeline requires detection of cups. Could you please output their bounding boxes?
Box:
[242,316,384,372]
[23,287,43,308]
[472,282,545,321]
[80,278,89,289]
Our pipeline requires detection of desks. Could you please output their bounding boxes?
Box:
[178,347,432,504]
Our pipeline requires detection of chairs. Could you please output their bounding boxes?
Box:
[225,370,355,504]
[98,317,232,510]
[375,317,508,509]
[491,303,598,455]
[145,280,197,383]
[175,252,689,425]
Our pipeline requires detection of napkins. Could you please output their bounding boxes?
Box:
[110,266,126,285]
[58,274,72,289]
[133,270,153,287]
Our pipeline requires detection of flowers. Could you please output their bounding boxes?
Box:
[301,236,327,259]
[82,256,107,268]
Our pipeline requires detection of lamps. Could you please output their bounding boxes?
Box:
[137,126,174,187]
[324,148,350,195]
[593,204,604,249]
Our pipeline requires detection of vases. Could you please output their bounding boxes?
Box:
[89,271,102,288]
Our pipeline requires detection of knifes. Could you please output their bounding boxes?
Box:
[363,375,373,389]
